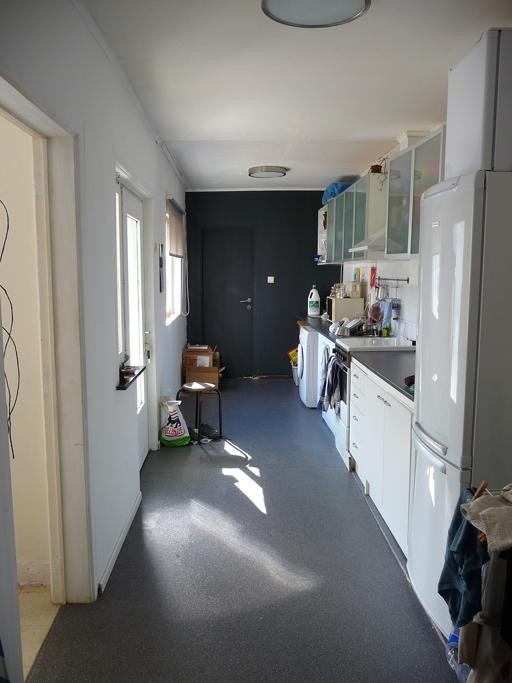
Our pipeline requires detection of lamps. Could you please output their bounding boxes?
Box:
[248,165,287,180]
[261,1,372,29]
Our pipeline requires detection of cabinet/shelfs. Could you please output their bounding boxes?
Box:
[387,129,445,254]
[343,174,366,262]
[312,205,327,263]
[327,192,343,262]
[370,374,415,557]
[347,355,370,496]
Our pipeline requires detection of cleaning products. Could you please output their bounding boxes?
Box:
[307,284,321,318]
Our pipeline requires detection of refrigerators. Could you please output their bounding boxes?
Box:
[407,170,511,641]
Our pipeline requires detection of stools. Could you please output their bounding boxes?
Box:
[175,380,222,441]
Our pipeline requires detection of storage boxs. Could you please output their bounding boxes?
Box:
[398,130,431,151]
[182,343,224,393]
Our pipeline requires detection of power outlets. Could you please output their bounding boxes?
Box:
[267,275,275,284]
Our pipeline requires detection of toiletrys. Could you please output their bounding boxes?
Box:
[382,323,391,337]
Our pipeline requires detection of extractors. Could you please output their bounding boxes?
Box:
[348,226,386,252]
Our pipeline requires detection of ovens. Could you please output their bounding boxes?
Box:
[329,347,351,427]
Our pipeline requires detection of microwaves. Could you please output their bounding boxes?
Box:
[326,297,365,323]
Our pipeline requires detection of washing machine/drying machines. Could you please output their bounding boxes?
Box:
[297,327,319,410]
